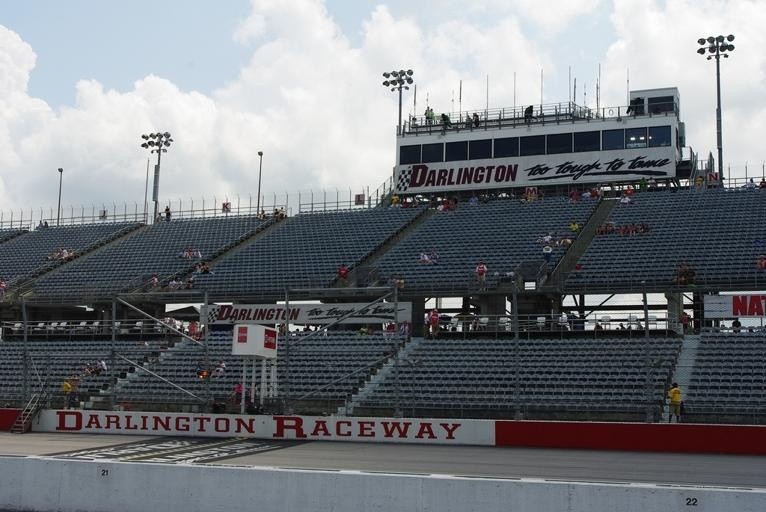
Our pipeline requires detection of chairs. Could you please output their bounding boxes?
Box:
[79,330,766,424]
[0,189,766,304]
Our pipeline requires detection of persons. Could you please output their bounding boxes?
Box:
[256,209,265,220]
[665,382,682,423]
[426,249,439,264]
[273,208,279,219]
[62,380,71,409]
[696,175,703,193]
[759,254,766,270]
[336,263,350,284]
[210,359,225,378]
[232,383,244,406]
[388,187,478,211]
[535,220,653,262]
[518,184,634,209]
[640,177,648,192]
[164,205,171,222]
[422,105,481,131]
[760,178,766,189]
[83,359,107,376]
[475,261,489,294]
[430,308,440,338]
[418,253,429,263]
[399,320,410,335]
[150,273,158,289]
[275,207,285,220]
[732,318,741,332]
[743,178,756,191]
[396,276,404,292]
[244,390,252,407]
[59,248,68,260]
[648,177,656,190]
[682,312,689,331]
[423,311,431,339]
[387,320,395,334]
[523,105,533,123]
[168,248,213,289]
[157,213,163,224]
[67,249,74,259]
[0,278,6,295]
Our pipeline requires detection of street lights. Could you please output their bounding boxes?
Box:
[56,168,65,226]
[382,70,413,132]
[697,34,735,185]
[141,132,173,222]
[256,152,265,216]
